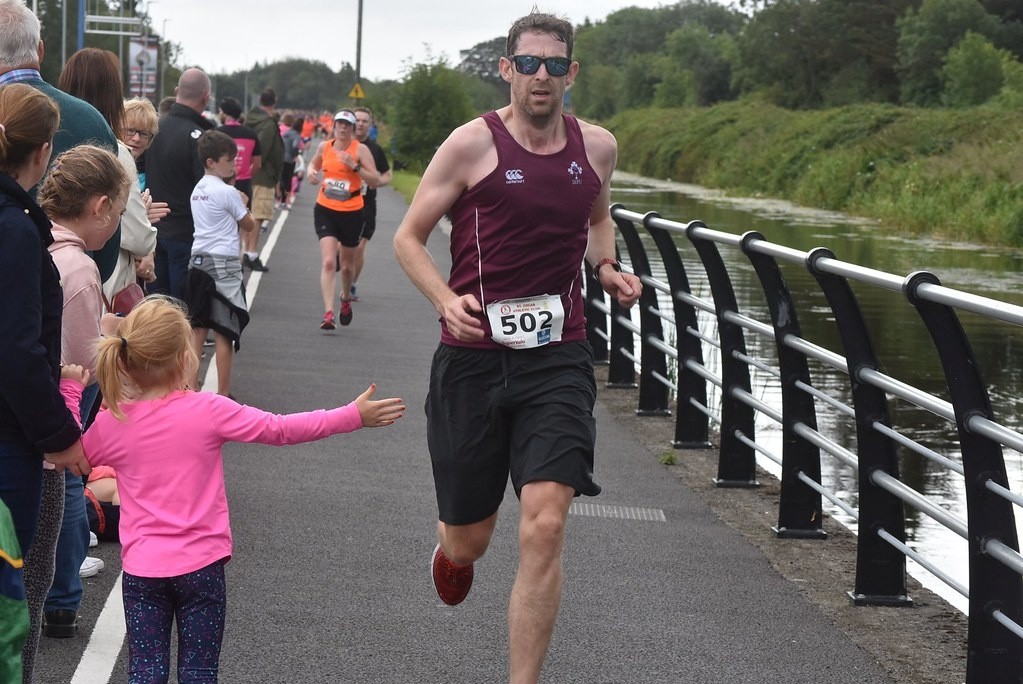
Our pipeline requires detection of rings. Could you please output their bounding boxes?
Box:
[145,269,150,275]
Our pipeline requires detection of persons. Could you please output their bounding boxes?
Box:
[392,12,643,684]
[336,106,392,302]
[0,1,172,684]
[201,89,306,273]
[184,126,254,404]
[306,109,380,332]
[297,108,378,150]
[44,292,406,684]
[145,66,231,359]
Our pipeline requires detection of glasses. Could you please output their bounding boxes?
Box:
[123,127,154,139]
[508,54,572,76]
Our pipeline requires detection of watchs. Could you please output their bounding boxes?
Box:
[354,164,361,172]
[592,258,623,283]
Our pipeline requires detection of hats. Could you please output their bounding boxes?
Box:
[334,111,357,124]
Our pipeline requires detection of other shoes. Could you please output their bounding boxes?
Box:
[202,338,216,347]
[90,531,98,547]
[42,608,79,635]
[86,556,105,569]
[79,559,98,578]
[350,288,360,301]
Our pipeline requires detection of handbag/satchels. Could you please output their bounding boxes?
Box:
[111,283,144,317]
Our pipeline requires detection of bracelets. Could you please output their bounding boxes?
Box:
[245,207,251,214]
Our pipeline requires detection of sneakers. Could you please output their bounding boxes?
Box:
[432,543,474,605]
[242,253,269,272]
[320,311,336,329]
[339,291,353,326]
